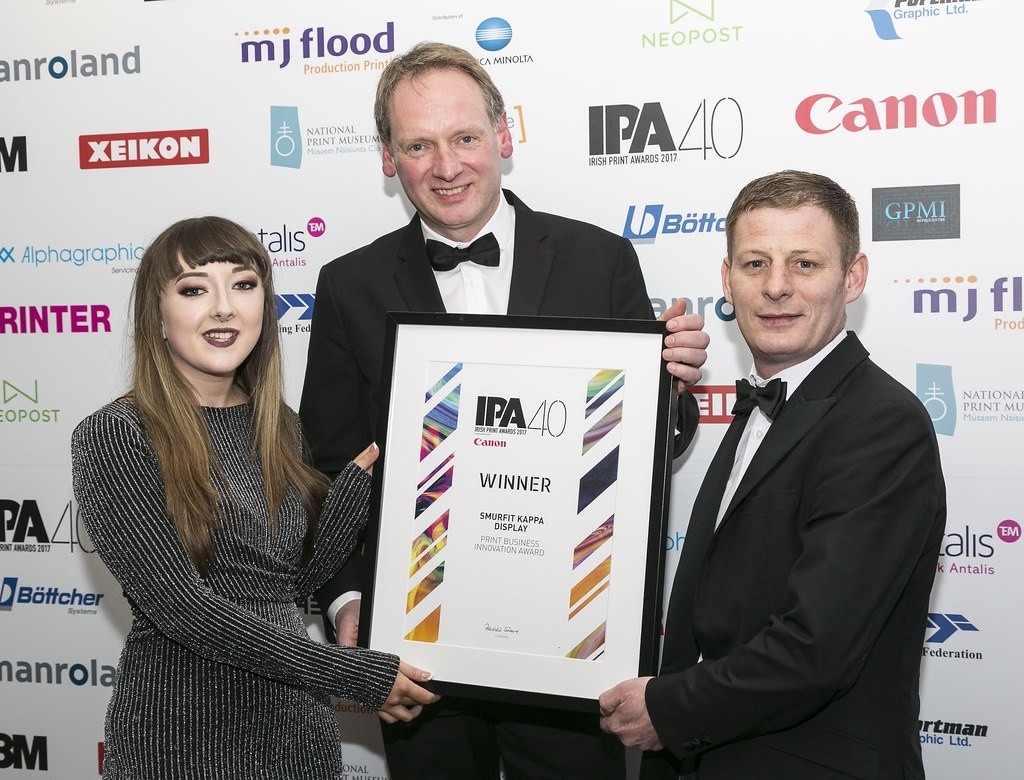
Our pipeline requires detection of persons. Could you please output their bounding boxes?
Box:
[599,169,948,780]
[298,42,711,780]
[70,216,441,780]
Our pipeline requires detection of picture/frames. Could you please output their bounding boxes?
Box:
[357,311,675,736]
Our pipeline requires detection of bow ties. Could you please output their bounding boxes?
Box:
[426,232,500,271]
[731,378,787,421]
